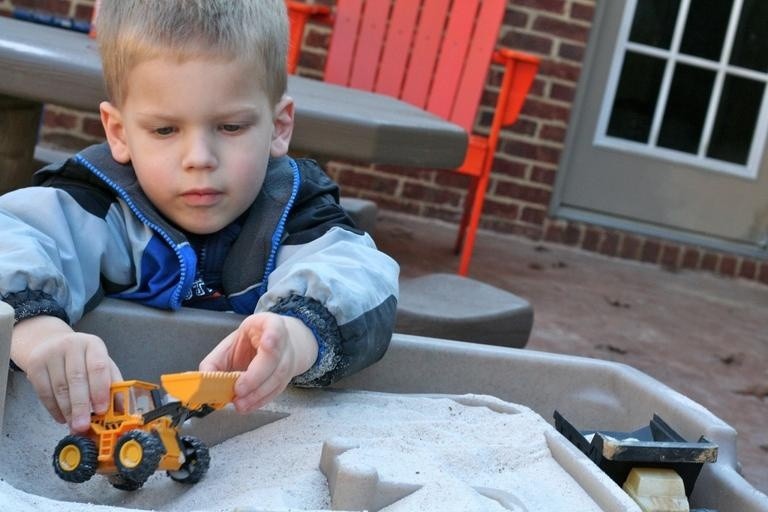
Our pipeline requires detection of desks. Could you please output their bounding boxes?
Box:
[0,14,469,199]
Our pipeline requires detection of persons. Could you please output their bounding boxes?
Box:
[0,0,399,433]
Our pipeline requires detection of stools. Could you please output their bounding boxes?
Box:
[389,271,534,349]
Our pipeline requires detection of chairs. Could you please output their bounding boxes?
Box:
[286,0,543,276]
